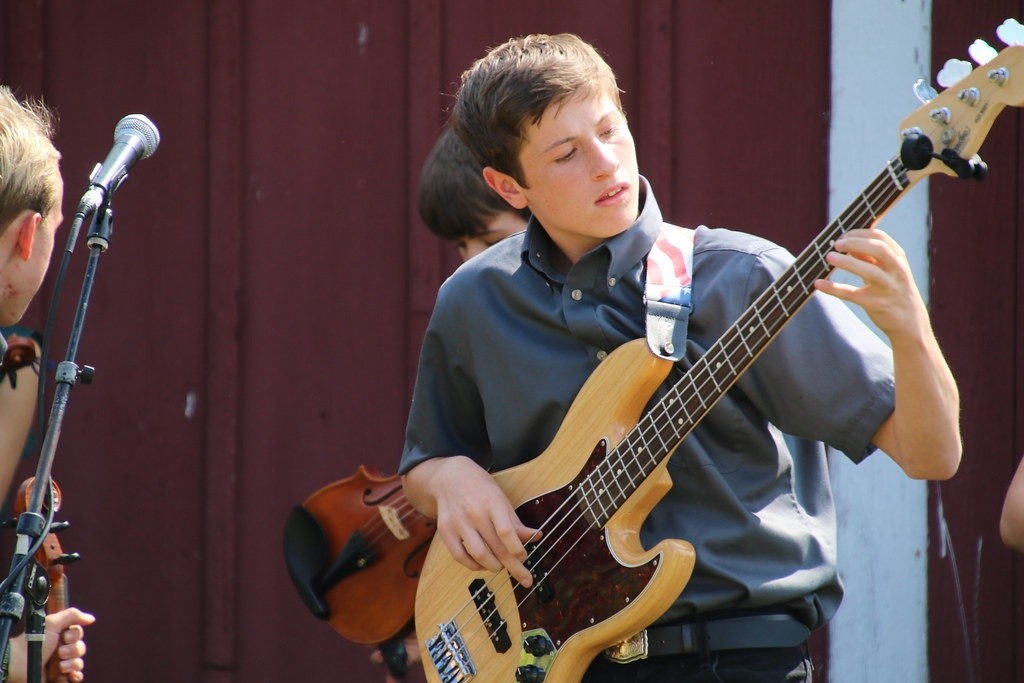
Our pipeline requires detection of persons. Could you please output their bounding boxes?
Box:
[397,32,962,683]
[0,80,96,682]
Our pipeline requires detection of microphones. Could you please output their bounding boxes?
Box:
[79,113,161,220]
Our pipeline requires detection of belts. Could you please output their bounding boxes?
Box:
[590,614,811,664]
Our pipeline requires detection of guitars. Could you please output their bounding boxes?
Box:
[415,16,1024,683]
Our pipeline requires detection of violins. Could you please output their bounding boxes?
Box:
[5,477,83,683]
[282,466,431,643]
